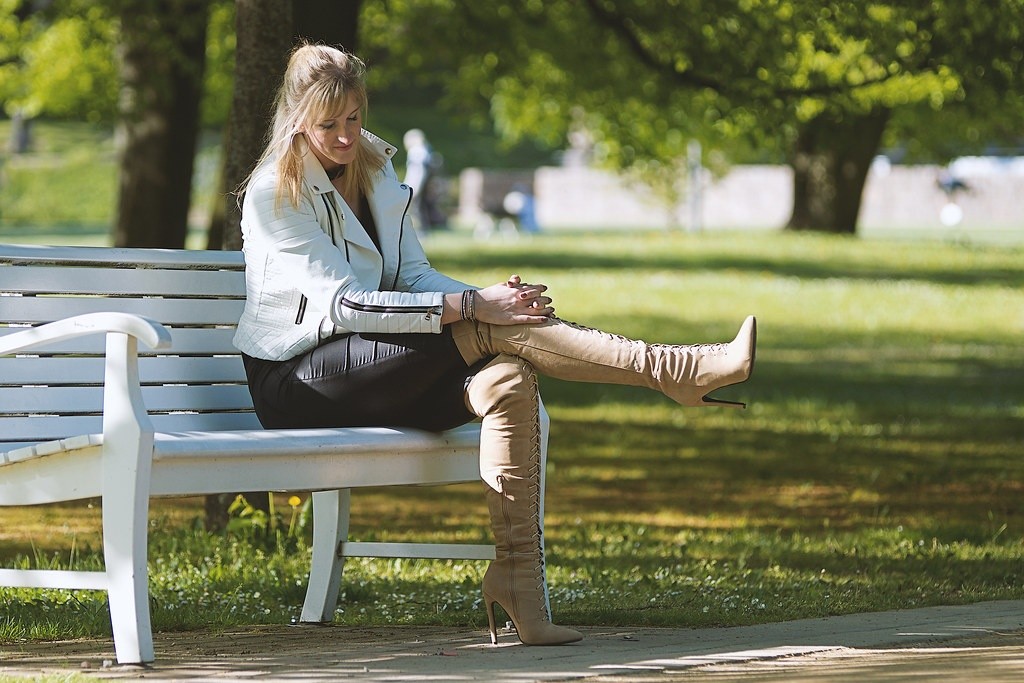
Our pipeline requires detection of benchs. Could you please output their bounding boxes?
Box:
[0,244,551,664]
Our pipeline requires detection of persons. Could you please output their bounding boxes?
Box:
[233,46,758,647]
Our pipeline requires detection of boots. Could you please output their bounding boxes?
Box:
[465,351,584,646]
[450,315,757,409]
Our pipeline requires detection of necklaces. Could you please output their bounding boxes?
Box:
[326,165,344,181]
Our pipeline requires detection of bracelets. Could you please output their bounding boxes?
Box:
[460,290,477,323]
